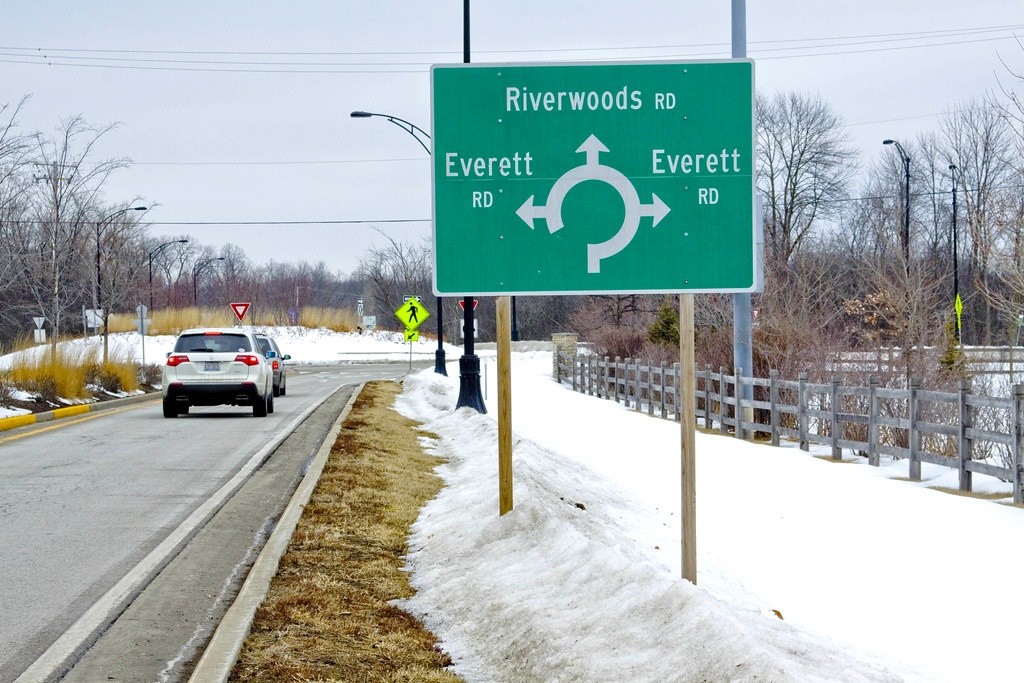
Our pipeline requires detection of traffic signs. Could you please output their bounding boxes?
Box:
[432,58,759,296]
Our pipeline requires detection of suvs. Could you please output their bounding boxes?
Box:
[162,327,276,418]
[254,333,292,398]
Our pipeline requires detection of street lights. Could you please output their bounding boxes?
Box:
[350,111,487,415]
[194,257,226,306]
[149,239,188,311]
[96,206,148,308]
[948,164,958,343]
[883,139,910,328]
